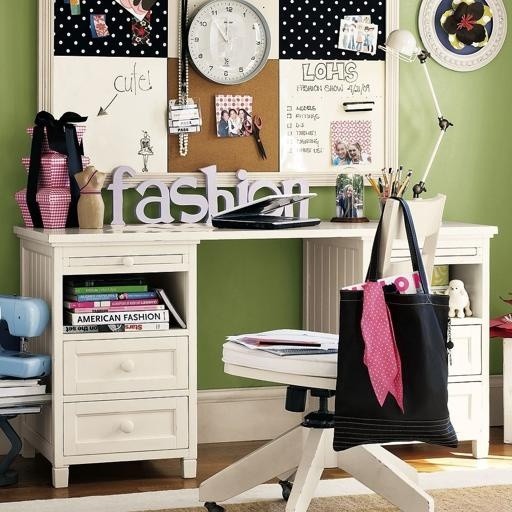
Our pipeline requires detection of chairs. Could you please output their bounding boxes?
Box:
[197,192,446,512]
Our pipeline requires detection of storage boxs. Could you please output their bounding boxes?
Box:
[14,122,90,229]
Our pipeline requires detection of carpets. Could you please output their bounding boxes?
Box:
[127,485,512,512]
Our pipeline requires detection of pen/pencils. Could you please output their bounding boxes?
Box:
[365,165,411,198]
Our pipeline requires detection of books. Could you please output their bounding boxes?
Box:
[0,393,52,407]
[63,277,186,335]
[0,378,42,387]
[0,405,42,413]
[0,384,47,398]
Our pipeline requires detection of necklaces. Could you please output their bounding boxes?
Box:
[178,0,189,157]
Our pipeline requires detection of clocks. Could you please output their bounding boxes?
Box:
[185,0,271,86]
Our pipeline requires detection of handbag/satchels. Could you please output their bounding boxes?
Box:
[333,196,458,452]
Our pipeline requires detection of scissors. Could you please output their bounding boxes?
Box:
[243,115,267,160]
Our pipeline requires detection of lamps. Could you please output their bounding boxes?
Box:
[378,29,454,198]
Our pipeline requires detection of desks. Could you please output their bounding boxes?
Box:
[12,221,499,490]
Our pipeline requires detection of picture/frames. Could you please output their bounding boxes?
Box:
[417,0,508,72]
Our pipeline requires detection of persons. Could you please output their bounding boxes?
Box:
[226,108,242,136]
[217,110,228,136]
[337,184,359,218]
[348,143,371,165]
[238,108,253,135]
[74,165,106,228]
[332,139,352,165]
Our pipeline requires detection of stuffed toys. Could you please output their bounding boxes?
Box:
[443,278,473,318]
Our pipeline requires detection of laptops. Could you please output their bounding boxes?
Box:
[211,192,321,228]
